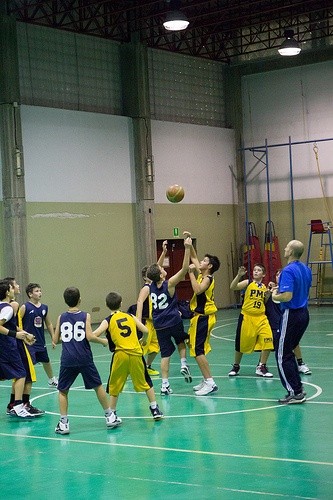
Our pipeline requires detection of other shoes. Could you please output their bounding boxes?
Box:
[287,393,306,403]
[279,393,292,402]
[298,360,312,374]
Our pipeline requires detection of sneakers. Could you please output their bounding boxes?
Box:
[55,417,69,434]
[149,404,163,419]
[255,363,274,377]
[105,412,115,429]
[48,376,58,387]
[6,407,11,415]
[193,380,207,390]
[10,403,35,417]
[28,404,45,415]
[107,410,122,427]
[147,365,159,376]
[195,380,217,396]
[228,363,240,376]
[161,382,173,395]
[180,364,192,383]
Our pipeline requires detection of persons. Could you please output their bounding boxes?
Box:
[259,268,311,374]
[4,276,45,416]
[146,237,193,395]
[18,283,59,387]
[182,231,220,396]
[270,240,313,403]
[53,287,122,435]
[92,292,164,419]
[135,241,167,376]
[0,279,33,417]
[228,263,273,376]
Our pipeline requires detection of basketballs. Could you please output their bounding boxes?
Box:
[166,184,185,203]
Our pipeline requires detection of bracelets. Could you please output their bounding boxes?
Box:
[7,330,16,338]
